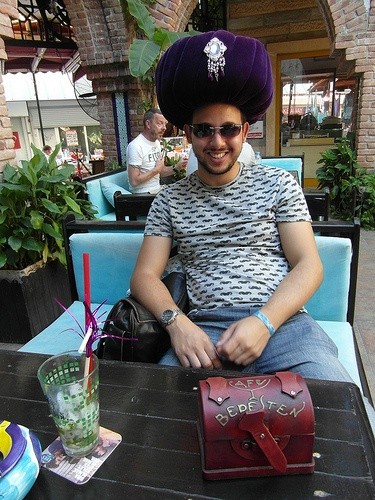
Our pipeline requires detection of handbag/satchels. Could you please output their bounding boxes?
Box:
[93,270,190,364]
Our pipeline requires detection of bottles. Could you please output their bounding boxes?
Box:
[255,152,262,163]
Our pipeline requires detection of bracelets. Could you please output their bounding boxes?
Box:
[173,164,178,172]
[252,311,275,337]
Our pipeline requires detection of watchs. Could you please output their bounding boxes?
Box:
[160,308,185,328]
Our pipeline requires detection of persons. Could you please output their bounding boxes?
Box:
[42,145,62,170]
[131,29,375,438]
[126,108,188,194]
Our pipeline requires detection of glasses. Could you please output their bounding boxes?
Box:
[187,122,244,139]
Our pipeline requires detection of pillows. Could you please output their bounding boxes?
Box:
[126,254,184,297]
[101,179,133,209]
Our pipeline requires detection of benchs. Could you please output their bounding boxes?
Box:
[256,152,305,189]
[114,187,330,222]
[17,214,374,409]
[72,165,129,223]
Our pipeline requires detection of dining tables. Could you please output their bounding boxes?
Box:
[0,347,375,500]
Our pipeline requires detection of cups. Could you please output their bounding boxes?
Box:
[37,350,99,458]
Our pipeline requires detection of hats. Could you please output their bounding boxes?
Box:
[156,29,275,130]
